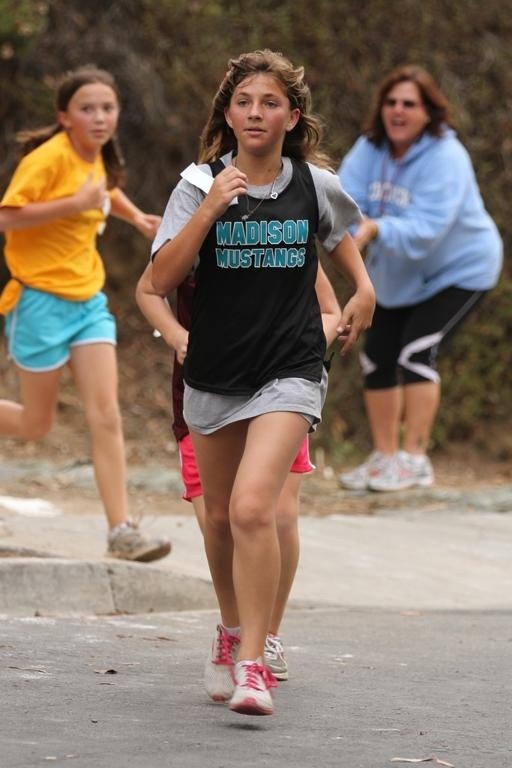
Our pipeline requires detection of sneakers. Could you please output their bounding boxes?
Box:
[108,522,171,562]
[204,624,288,715]
[338,449,434,492]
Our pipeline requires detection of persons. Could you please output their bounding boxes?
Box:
[151,47,377,715]
[132,238,341,683]
[328,65,502,493]
[1,69,172,562]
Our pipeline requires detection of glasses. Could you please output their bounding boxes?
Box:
[385,99,416,108]
